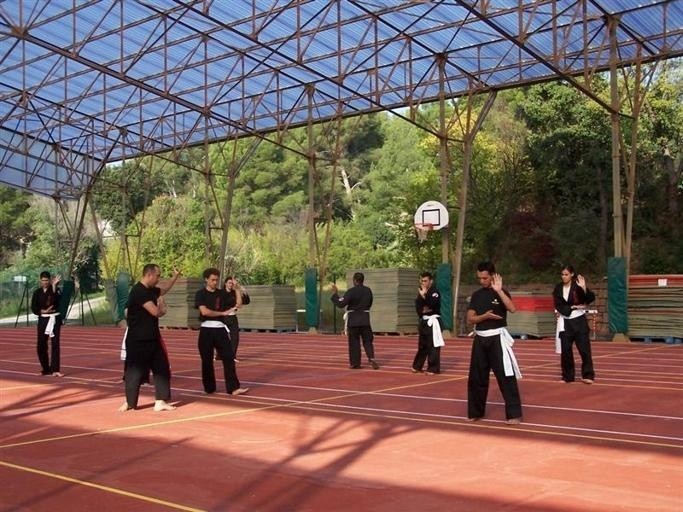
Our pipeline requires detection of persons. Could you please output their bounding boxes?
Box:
[32,271,65,378]
[118,264,250,411]
[464,261,521,426]
[331,272,379,369]
[411,273,444,374]
[554,265,596,385]
